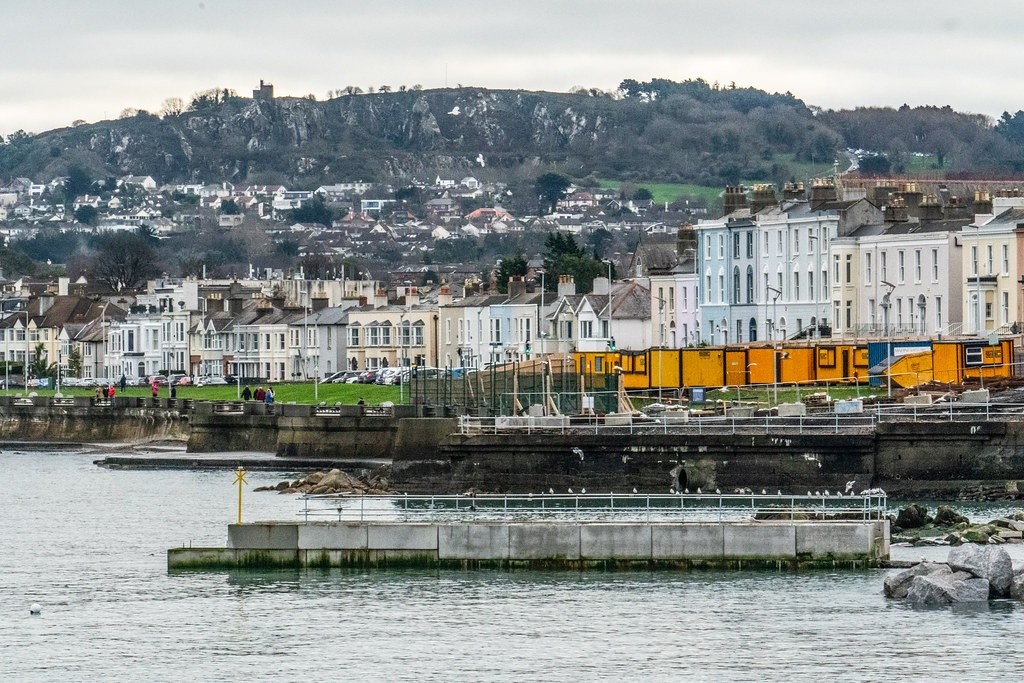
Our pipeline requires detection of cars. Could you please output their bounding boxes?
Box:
[0,373,241,388]
[318,361,519,386]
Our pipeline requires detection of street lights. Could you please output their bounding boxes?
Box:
[6,310,28,395]
[403,281,412,370]
[197,296,204,377]
[300,291,308,385]
[535,270,545,338]
[967,222,980,330]
[686,247,697,346]
[96,305,107,377]
[808,235,819,340]
[601,259,612,338]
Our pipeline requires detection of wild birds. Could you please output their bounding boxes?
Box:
[475,152,486,169]
[446,106,461,117]
[28,603,42,615]
[609,351,876,423]
[404,436,887,518]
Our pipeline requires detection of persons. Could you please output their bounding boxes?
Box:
[120,374,126,392]
[95,385,101,397]
[171,388,176,397]
[241,385,275,403]
[358,398,364,404]
[103,384,116,397]
[152,384,158,397]
[145,377,149,384]
